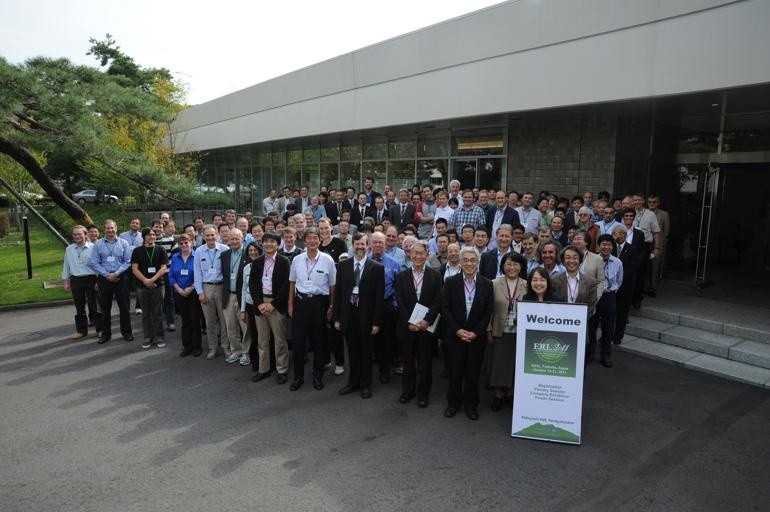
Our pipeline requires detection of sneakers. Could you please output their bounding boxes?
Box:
[223,350,259,369]
[207,351,217,360]
[324,363,344,375]
[98,332,110,344]
[126,333,133,341]
[143,338,166,348]
[135,309,142,314]
[71,331,87,340]
[441,369,448,378]
[169,324,175,330]
[379,367,404,384]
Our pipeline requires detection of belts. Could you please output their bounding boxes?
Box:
[229,291,237,294]
[202,281,222,284]
[263,294,273,298]
[306,294,314,298]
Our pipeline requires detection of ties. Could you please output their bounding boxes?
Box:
[350,199,353,207]
[338,203,340,212]
[379,210,380,223]
[402,205,405,216]
[361,209,363,218]
[351,263,360,305]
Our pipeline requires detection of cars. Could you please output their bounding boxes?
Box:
[193,182,256,196]
[71,190,119,206]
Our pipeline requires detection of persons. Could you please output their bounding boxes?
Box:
[61,178,675,421]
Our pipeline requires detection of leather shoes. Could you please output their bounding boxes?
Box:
[417,397,429,407]
[339,386,358,395]
[464,407,478,419]
[491,395,512,412]
[181,350,202,357]
[252,373,265,382]
[314,377,323,389]
[648,291,657,298]
[445,407,455,417]
[633,305,641,309]
[599,357,613,368]
[362,389,371,398]
[614,341,621,345]
[399,390,416,403]
[290,376,304,390]
[277,375,288,383]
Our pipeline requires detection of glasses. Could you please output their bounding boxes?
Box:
[180,241,188,245]
